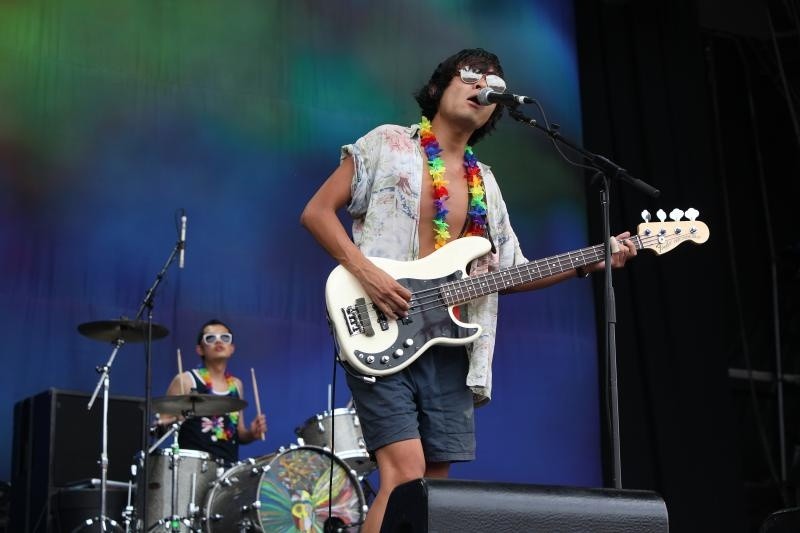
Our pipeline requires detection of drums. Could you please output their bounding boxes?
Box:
[202,443,368,533]
[132,447,217,533]
[298,407,371,473]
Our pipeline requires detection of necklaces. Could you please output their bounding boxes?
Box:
[195,363,239,442]
[418,116,488,319]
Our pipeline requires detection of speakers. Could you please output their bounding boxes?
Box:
[9,488,143,533]
[378,478,669,533]
[11,387,148,488]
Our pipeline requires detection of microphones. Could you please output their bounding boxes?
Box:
[177,214,189,271]
[476,86,535,106]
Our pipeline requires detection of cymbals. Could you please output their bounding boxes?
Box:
[77,318,169,342]
[150,394,248,416]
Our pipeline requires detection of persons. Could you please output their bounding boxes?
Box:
[300,48,637,533]
[159,319,266,478]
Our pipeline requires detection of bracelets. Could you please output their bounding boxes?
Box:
[576,265,592,280]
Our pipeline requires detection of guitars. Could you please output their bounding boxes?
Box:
[324,208,712,376]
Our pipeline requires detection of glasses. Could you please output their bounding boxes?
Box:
[203,333,233,345]
[459,66,506,93]
